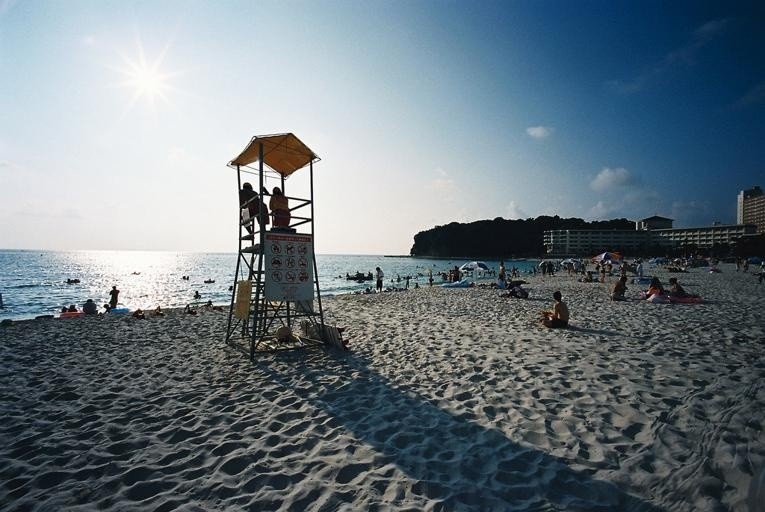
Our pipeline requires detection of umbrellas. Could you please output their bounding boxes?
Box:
[590,250,621,267]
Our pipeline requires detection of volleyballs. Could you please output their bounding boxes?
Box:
[276,327,293,338]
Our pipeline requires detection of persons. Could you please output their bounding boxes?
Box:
[194,291,201,299]
[270,187,291,227]
[343,261,727,301]
[108,286,119,309]
[758,261,765,283]
[184,304,196,315]
[541,291,569,328]
[240,183,269,234]
[103,304,113,314]
[66,279,80,283]
[734,256,749,273]
[62,299,98,312]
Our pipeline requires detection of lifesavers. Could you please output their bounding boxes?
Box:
[60,311,85,319]
[110,308,130,314]
